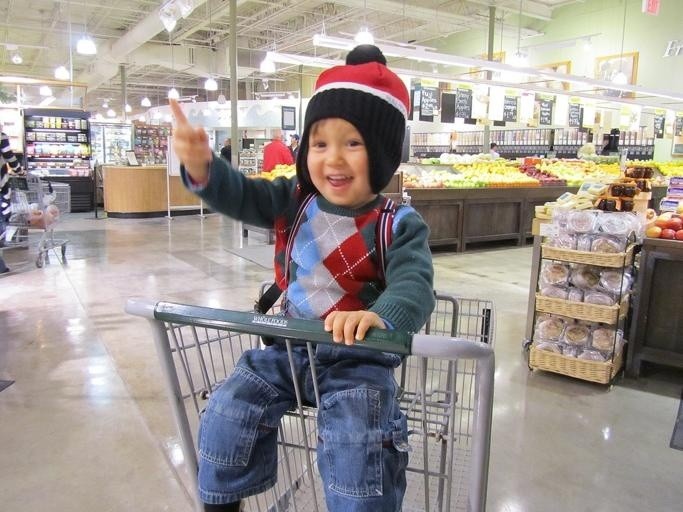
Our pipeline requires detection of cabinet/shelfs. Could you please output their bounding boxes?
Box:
[133,120,173,164]
[23,108,93,212]
[90,122,132,219]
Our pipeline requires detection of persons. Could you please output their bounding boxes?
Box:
[491,143,499,158]
[286,133,300,163]
[0,123,28,276]
[168,43,437,512]
[262,128,294,172]
[220,138,231,161]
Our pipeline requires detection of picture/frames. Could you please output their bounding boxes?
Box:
[282,106,295,130]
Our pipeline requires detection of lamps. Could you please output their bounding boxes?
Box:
[12,1,219,119]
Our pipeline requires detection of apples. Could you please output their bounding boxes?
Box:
[646,217,683,241]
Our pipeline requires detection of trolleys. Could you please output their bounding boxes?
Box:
[121,278,496,512]
[0,170,71,268]
